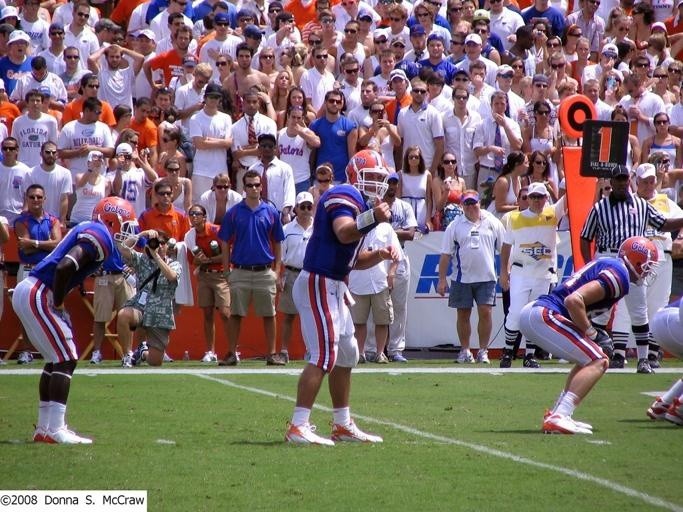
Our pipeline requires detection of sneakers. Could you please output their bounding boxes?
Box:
[285,421,335,446]
[202,350,240,365]
[162,353,173,363]
[500,353,552,367]
[332,420,383,442]
[267,352,288,365]
[543,408,593,435]
[357,352,408,363]
[90,350,103,364]
[647,395,682,424]
[609,352,662,373]
[456,349,489,364]
[121,341,148,368]
[17,352,33,364]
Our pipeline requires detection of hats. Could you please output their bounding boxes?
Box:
[0,78,6,94]
[651,22,667,33]
[527,183,548,196]
[39,87,51,97]
[676,0,683,8]
[295,192,314,204]
[94,18,156,41]
[258,133,276,144]
[461,190,480,202]
[386,172,400,181]
[117,143,133,155]
[612,151,670,179]
[1,7,31,47]
[87,151,104,165]
[601,44,618,59]
[78,73,98,95]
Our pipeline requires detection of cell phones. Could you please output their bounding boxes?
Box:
[377,110,383,128]
[123,154,132,159]
[386,80,392,91]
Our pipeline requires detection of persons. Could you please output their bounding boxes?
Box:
[647,295,683,424]
[519,235,662,434]
[0,1,682,373]
[11,196,140,444]
[285,149,398,444]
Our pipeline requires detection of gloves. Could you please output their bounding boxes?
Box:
[52,307,72,328]
[586,326,614,359]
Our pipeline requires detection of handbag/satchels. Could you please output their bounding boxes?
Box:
[440,202,464,230]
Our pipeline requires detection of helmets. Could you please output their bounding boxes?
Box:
[345,150,389,199]
[92,197,139,250]
[618,236,659,287]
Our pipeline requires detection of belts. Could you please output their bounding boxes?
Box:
[94,270,122,276]
[285,265,302,272]
[664,250,672,253]
[232,263,272,271]
[199,268,224,273]
[598,248,619,253]
[480,164,496,170]
[513,262,524,267]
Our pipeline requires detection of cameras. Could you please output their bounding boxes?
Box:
[340,83,345,89]
[143,148,151,156]
[146,237,159,260]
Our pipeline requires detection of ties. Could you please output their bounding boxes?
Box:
[494,121,502,172]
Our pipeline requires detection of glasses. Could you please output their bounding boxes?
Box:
[317,180,331,183]
[88,84,99,89]
[50,12,89,58]
[636,63,681,77]
[521,194,546,199]
[300,205,312,211]
[2,147,16,151]
[93,110,101,115]
[612,14,630,32]
[45,151,56,154]
[534,161,546,165]
[607,78,620,82]
[131,138,273,216]
[29,196,42,199]
[464,200,478,205]
[655,121,669,124]
[442,160,456,165]
[408,154,419,159]
